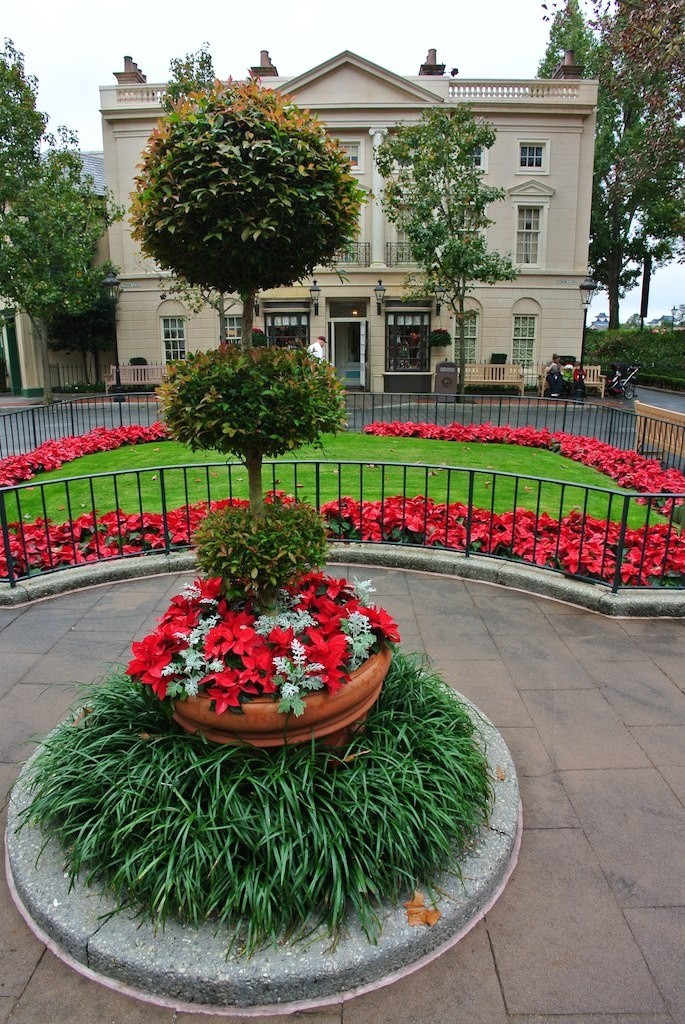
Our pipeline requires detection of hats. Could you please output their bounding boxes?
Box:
[319,336,327,343]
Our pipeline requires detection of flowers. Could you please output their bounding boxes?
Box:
[252,328,267,347]
[124,569,402,717]
[427,328,452,348]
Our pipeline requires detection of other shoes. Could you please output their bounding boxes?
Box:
[551,393,560,397]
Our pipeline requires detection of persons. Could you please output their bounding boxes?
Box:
[306,336,328,365]
[545,354,587,398]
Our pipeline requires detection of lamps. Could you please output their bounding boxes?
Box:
[309,279,321,316]
[374,278,387,315]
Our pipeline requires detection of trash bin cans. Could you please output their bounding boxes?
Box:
[434,362,458,402]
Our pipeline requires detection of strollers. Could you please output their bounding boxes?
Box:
[605,362,642,400]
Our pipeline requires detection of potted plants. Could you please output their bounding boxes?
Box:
[122,69,395,767]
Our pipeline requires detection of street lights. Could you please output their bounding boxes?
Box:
[670,306,679,331]
[102,271,128,402]
[574,277,598,405]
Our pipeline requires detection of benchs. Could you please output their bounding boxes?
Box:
[538,365,607,398]
[103,364,167,394]
[634,400,685,463]
[464,364,525,396]
